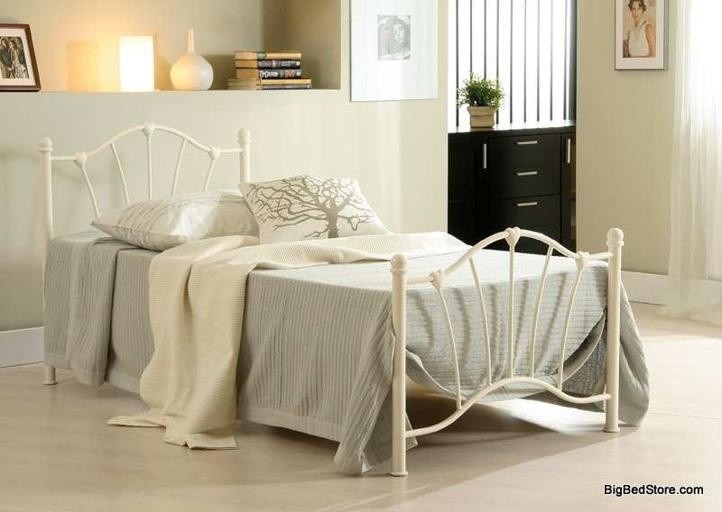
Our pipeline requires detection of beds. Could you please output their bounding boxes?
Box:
[36,123,625,477]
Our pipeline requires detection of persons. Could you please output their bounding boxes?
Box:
[0,39,15,78]
[9,40,28,78]
[623,0,656,57]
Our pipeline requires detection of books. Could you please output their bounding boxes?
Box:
[228,51,312,90]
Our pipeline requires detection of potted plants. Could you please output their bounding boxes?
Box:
[454,72,505,128]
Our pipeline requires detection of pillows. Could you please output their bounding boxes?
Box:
[88,173,394,254]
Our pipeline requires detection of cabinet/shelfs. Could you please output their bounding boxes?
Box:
[446,125,577,259]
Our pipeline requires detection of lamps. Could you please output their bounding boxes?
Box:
[118,34,157,94]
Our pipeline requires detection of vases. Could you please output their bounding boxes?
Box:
[169,28,215,92]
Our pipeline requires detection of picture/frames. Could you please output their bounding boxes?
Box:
[1,22,43,93]
[613,0,671,72]
[348,0,440,103]
